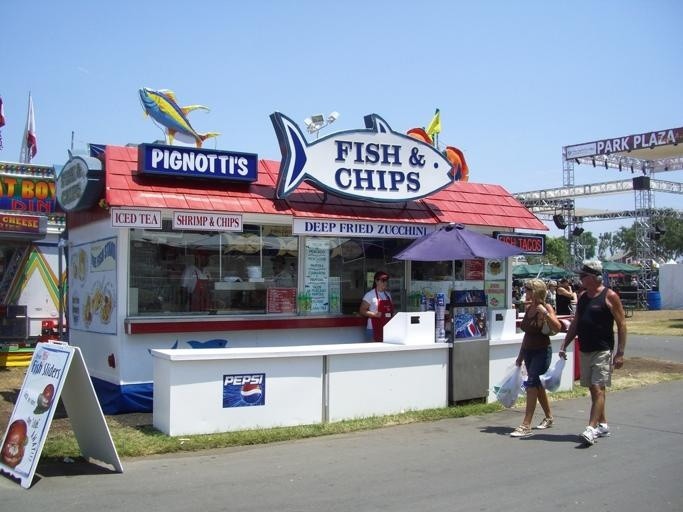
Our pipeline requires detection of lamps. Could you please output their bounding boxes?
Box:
[553,213,566,229]
[575,156,647,176]
[653,222,665,236]
[571,226,584,237]
[647,229,661,240]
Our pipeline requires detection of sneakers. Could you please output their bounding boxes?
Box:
[537,416,553,429]
[510,426,532,437]
[579,423,609,445]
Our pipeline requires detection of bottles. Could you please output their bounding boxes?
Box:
[477,312,486,335]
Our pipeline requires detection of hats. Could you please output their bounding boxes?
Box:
[572,265,601,277]
[375,271,389,280]
[195,249,212,257]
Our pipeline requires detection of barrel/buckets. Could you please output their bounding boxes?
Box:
[647,291,661,310]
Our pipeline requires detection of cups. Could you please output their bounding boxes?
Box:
[419,293,446,343]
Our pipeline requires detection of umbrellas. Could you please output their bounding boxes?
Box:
[392,223,526,290]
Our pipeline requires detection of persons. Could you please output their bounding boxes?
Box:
[179,245,216,312]
[559,260,627,445]
[269,256,294,288]
[511,278,588,315]
[631,276,637,286]
[360,271,395,343]
[510,278,562,436]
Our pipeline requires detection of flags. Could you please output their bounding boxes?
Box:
[426,109,440,136]
[19,95,37,163]
[0,95,6,153]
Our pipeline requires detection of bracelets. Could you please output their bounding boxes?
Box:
[616,352,624,356]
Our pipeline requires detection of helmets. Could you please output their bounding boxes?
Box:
[270,255,285,264]
